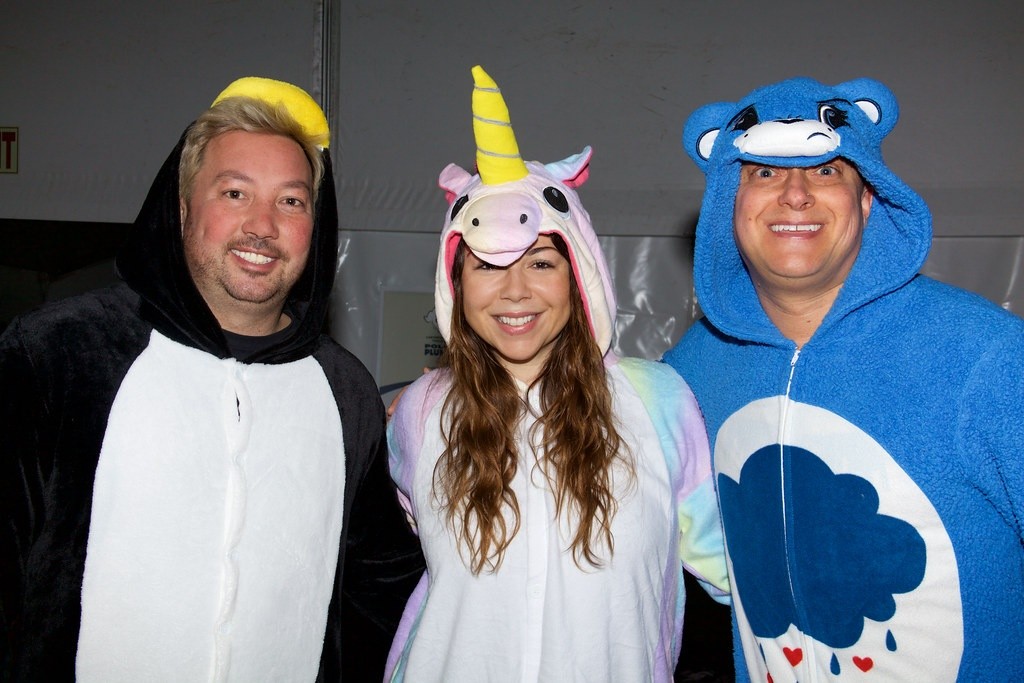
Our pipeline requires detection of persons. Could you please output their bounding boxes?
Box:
[386,74,1022,683]
[1,71,423,683]
[372,62,739,683]
